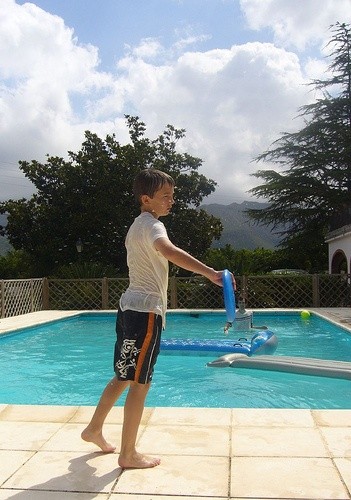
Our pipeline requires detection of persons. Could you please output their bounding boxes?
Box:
[81,168,237,469]
[223,294,268,334]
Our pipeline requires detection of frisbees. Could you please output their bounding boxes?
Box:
[223,270,235,323]
